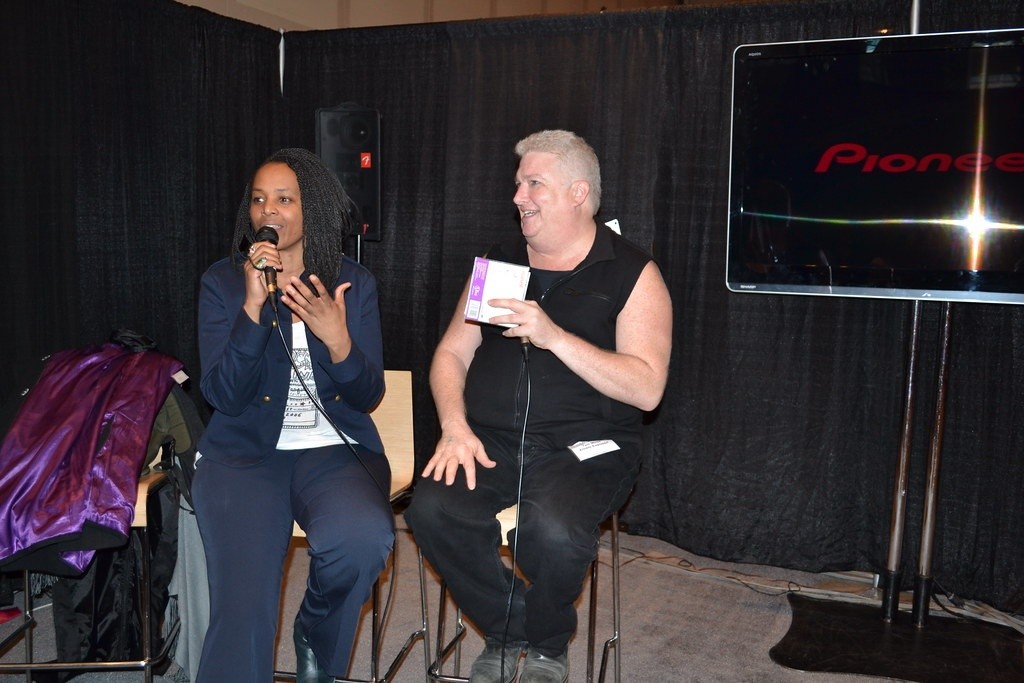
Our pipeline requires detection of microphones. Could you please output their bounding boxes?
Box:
[520,332,532,364]
[256,226,277,307]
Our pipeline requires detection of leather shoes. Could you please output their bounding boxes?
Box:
[468,635,522,683]
[519,643,570,683]
[293,609,334,683]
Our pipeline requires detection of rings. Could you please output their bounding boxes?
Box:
[256,257,266,269]
[248,246,255,253]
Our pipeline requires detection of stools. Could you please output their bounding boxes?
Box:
[0,352,211,683]
[274,370,621,683]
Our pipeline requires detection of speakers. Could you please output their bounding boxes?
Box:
[315,103,381,242]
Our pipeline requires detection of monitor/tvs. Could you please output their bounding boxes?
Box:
[725,27,1024,306]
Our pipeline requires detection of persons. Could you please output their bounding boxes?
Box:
[188,148,395,683]
[401,129,674,683]
